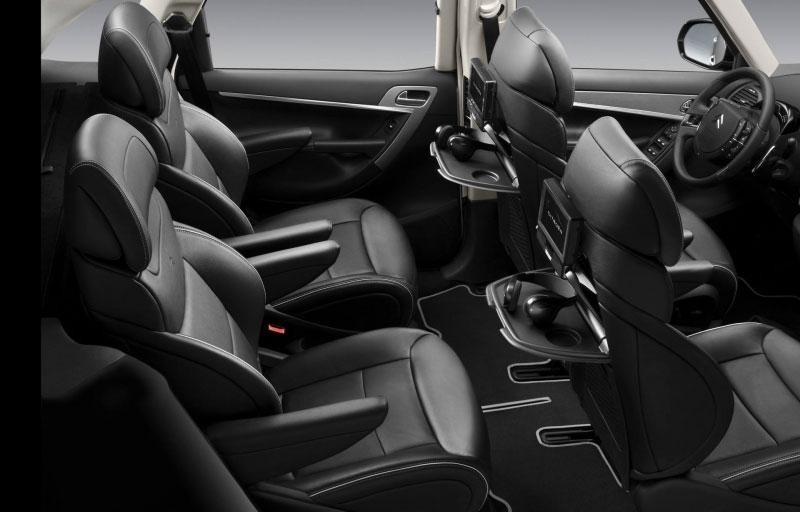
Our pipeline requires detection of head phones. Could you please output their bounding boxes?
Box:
[502,268,581,327]
[436,121,502,160]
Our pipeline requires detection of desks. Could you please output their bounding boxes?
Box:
[430,131,519,191]
[486,267,610,365]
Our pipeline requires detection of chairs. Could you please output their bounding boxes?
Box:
[484,7,737,328]
[54,113,492,509]
[561,116,800,512]
[48,1,492,352]
